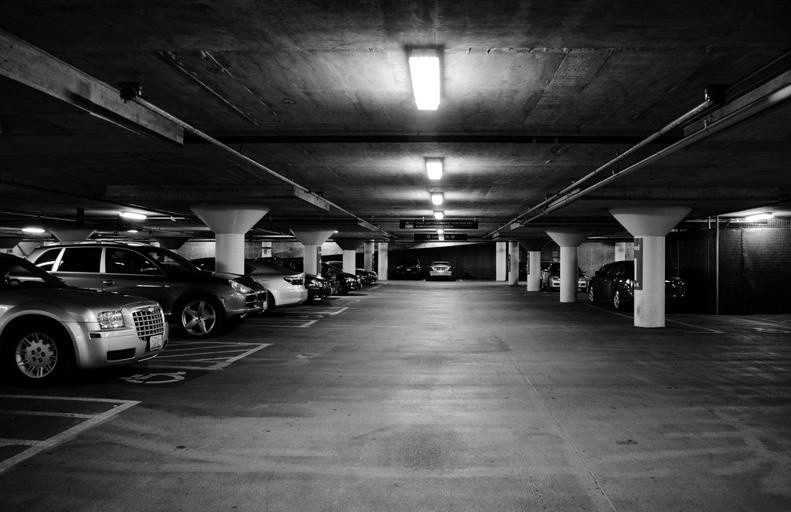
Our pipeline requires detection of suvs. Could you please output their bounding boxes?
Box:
[4,236,270,339]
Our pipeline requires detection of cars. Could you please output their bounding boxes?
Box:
[0,252,170,386]
[587,259,688,309]
[392,255,455,277]
[541,260,588,292]
[190,257,378,313]
